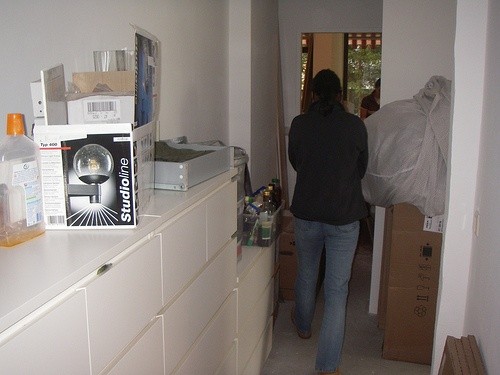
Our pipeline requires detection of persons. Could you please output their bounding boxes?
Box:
[288,67,369,375]
[360,78,381,118]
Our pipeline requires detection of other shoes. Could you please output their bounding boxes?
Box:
[319,369,340,375]
[290,306,312,339]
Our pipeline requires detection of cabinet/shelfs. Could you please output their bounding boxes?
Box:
[0,166,280,375]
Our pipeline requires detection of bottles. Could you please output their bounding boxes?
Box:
[243,177,281,245]
[0,113,47,248]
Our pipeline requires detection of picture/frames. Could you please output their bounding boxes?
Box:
[438,335,487,375]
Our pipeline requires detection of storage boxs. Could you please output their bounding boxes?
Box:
[278,140,448,365]
[240,200,286,247]
[33,23,162,231]
